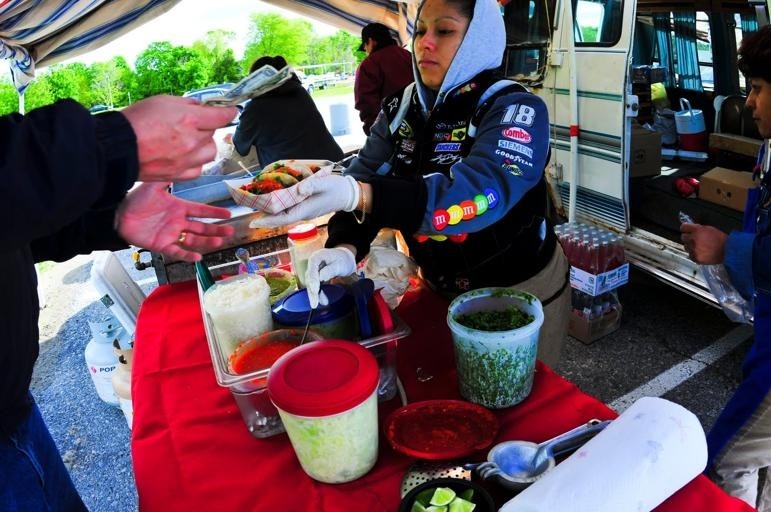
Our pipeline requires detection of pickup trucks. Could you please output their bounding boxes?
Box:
[293,69,338,91]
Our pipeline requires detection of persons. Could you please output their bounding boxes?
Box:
[233,55,344,169]
[678,24,770,512]
[0,91,239,512]
[248,0,572,376]
[350,23,418,136]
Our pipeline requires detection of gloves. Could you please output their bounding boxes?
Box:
[305,247,358,309]
[249,174,359,229]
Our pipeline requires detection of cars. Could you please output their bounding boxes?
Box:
[90,103,109,112]
[182,85,244,103]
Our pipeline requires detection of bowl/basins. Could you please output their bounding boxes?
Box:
[230,328,325,418]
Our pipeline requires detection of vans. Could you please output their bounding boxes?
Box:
[492,0,769,326]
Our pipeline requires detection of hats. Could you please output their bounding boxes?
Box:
[358,23,389,51]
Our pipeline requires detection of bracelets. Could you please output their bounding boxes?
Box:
[352,180,366,224]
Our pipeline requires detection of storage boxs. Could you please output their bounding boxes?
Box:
[629,123,664,178]
[697,132,763,212]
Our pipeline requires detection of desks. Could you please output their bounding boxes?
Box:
[133,253,758,512]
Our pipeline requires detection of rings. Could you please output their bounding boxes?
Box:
[177,232,186,246]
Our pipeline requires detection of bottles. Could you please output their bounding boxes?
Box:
[286,223,325,290]
[551,219,630,345]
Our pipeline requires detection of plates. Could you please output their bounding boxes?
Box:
[384,397,499,461]
